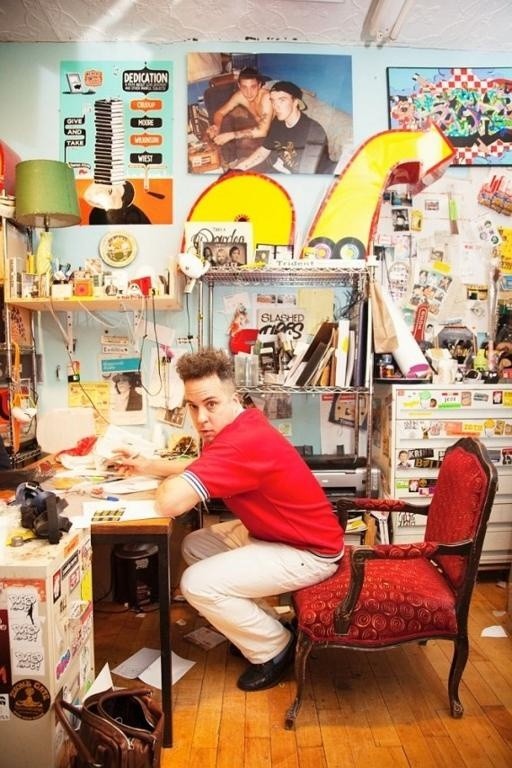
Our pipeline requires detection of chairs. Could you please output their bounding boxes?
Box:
[283,437,498,729]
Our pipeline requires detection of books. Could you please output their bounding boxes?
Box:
[278,312,368,389]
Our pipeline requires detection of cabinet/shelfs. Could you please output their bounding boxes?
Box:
[196,237,376,545]
[0,530,96,768]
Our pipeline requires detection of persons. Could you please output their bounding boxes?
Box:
[430,248,445,265]
[409,266,451,315]
[204,65,286,172]
[102,347,346,691]
[110,372,144,413]
[203,241,246,268]
[391,209,407,231]
[397,450,413,468]
[233,78,338,174]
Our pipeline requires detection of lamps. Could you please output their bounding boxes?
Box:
[14,160,81,297]
[367,1,414,40]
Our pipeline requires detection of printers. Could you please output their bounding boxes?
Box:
[302,454,379,505]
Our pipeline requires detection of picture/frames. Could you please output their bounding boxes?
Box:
[201,242,293,265]
[433,323,479,373]
[99,230,138,268]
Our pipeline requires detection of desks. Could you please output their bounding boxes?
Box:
[375,384,512,572]
[1,455,203,748]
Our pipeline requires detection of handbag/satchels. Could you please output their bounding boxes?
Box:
[54,686,165,767]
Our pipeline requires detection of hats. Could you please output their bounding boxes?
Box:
[271,81,307,111]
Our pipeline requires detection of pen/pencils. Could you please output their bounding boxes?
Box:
[91,493,119,501]
[114,453,139,470]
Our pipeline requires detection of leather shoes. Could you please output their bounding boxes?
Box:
[230,620,295,691]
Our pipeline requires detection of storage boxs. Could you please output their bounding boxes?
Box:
[188,145,222,173]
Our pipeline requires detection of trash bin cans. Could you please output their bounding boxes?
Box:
[111,542,159,606]
